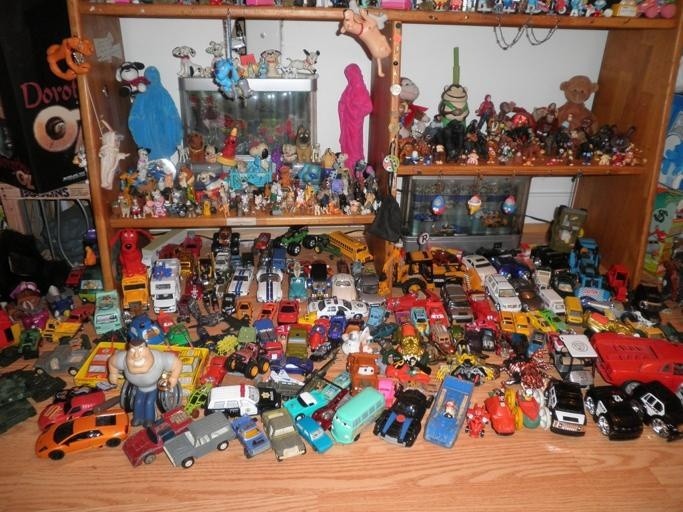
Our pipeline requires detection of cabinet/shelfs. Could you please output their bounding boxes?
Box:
[65,1,682,292]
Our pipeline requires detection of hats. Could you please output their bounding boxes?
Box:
[366,194,404,242]
